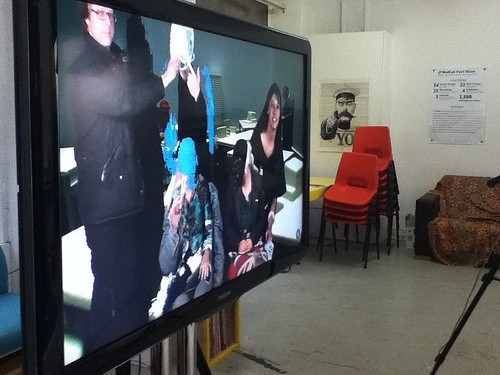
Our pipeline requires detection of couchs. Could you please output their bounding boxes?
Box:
[415,175,500,256]
[0,245,21,358]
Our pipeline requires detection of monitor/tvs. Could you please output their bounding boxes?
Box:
[12,0,313,375]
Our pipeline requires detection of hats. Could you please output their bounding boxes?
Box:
[332,86,361,99]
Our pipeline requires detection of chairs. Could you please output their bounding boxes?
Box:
[317,126,401,269]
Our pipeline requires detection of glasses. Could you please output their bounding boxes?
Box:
[88,8,119,22]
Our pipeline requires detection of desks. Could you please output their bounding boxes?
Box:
[218,120,301,245]
[60,146,172,318]
[296,176,335,202]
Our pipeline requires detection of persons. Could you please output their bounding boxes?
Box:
[157,136,225,313]
[278,85,297,151]
[248,82,283,243]
[216,137,274,282]
[172,41,215,183]
[60,0,181,359]
